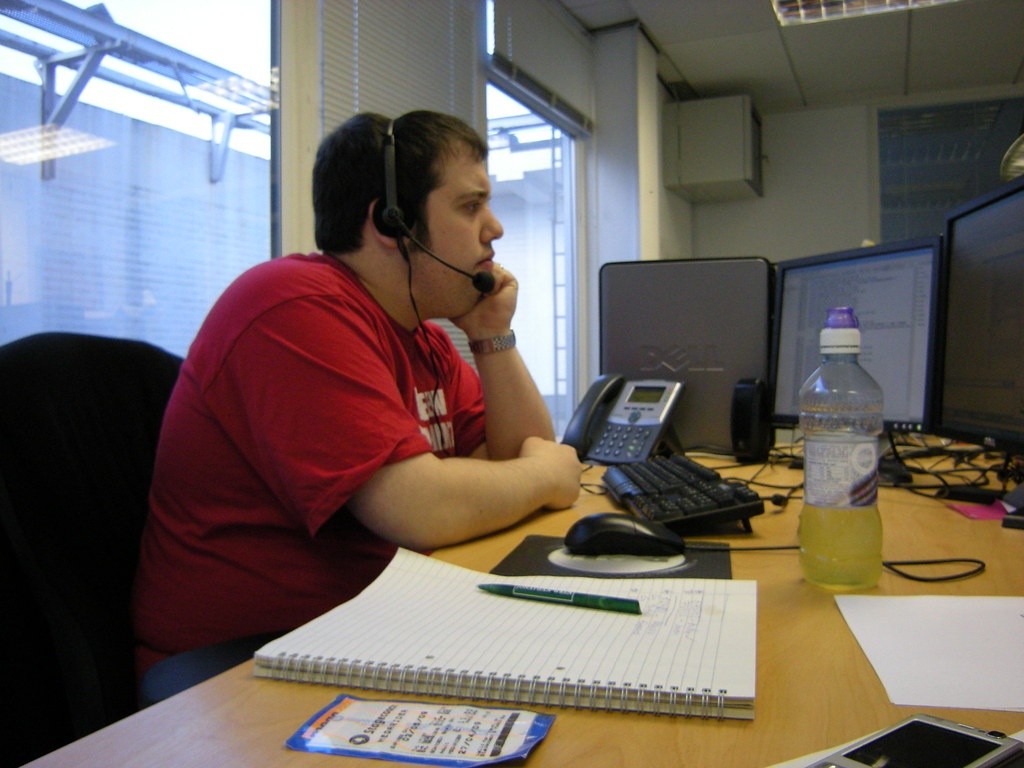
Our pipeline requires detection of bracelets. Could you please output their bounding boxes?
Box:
[468,329,516,354]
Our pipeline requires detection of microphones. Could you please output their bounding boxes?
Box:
[394,219,494,293]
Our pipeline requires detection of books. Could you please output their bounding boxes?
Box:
[253,546,758,728]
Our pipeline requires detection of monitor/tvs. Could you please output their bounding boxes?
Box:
[762,232,941,440]
[926,170,1023,514]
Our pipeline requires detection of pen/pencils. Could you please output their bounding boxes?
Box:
[476,582,643,615]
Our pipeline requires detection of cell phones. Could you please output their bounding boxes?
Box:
[799,715,1023,767]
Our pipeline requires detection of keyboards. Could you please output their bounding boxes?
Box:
[600,451,764,538]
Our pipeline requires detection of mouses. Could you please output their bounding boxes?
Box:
[560,510,685,555]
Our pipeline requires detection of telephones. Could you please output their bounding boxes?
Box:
[560,369,687,466]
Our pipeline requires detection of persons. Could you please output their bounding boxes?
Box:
[134,111,582,713]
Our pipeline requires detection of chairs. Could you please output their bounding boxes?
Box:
[0,331,187,768]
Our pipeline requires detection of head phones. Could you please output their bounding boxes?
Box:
[371,117,413,236]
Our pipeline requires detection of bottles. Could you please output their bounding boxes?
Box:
[798,305,884,589]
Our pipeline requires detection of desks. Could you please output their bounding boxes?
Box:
[18,427,1024,768]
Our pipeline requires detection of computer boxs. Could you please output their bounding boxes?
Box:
[593,256,774,453]
